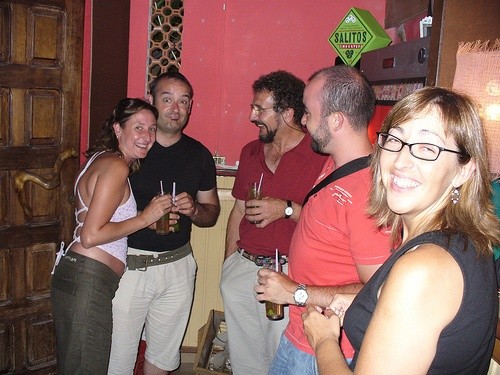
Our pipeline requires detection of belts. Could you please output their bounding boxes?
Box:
[237,247,288,266]
[126,240,193,271]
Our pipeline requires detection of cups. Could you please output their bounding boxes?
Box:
[263,263,283,320]
[155,191,179,235]
[248,181,263,224]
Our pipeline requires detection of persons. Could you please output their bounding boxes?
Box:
[51,97,159,375]
[107,71,221,375]
[220,66,500,375]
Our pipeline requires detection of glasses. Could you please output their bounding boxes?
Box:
[250,103,276,113]
[376,131,463,162]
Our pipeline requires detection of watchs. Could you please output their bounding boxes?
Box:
[293,283,308,307]
[284,200,293,219]
